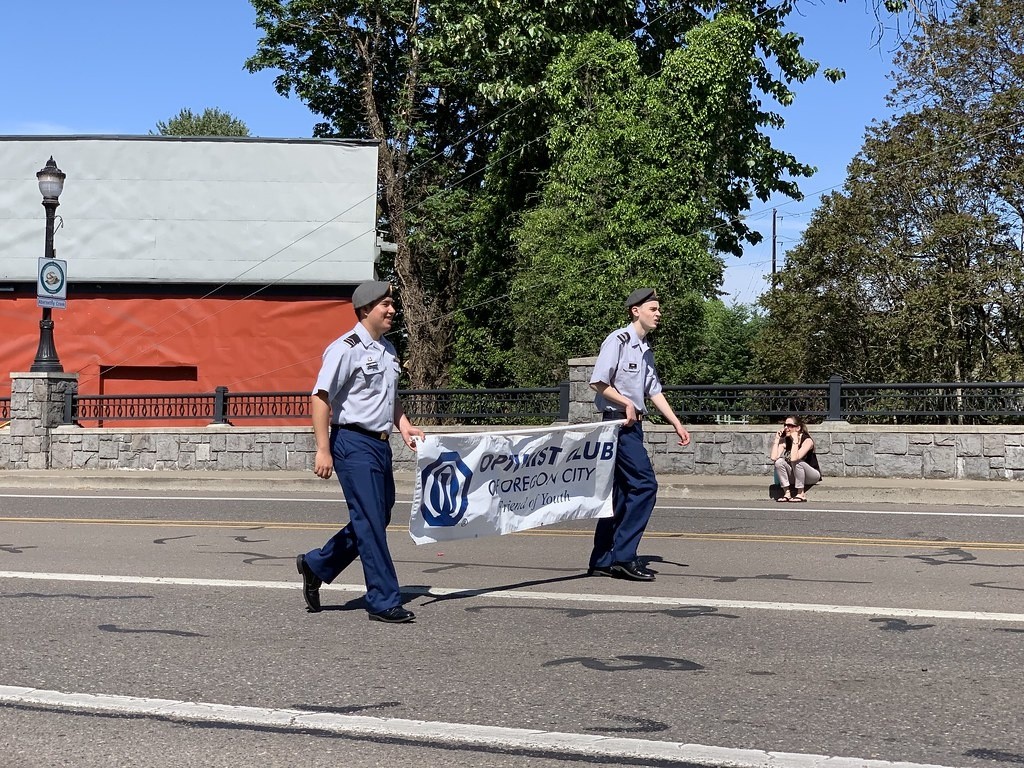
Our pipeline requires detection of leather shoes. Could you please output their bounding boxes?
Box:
[297,554,321,613]
[369,606,415,623]
[588,560,656,580]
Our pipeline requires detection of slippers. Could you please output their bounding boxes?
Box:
[776,497,808,502]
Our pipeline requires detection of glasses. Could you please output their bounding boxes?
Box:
[784,422,798,428]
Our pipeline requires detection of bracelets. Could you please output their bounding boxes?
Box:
[793,442,798,444]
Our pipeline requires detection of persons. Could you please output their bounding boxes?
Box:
[771,415,822,502]
[296,280,426,623]
[586,288,691,580]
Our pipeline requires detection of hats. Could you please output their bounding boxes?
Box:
[624,288,656,306]
[352,281,392,310]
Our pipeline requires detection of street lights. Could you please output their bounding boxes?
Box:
[27,154,70,371]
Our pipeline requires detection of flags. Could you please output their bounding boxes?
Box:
[410,425,619,545]
